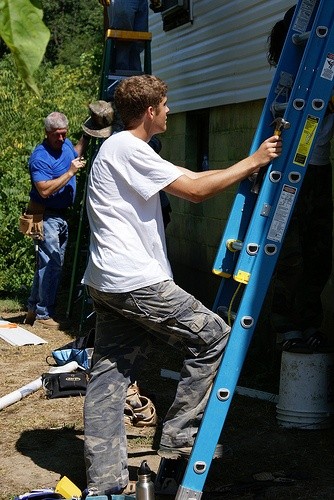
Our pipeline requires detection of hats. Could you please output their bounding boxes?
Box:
[81,100,114,137]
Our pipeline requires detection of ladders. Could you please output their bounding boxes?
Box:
[64,29,156,328]
[176,0,334,500]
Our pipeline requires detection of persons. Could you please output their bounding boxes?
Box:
[82,100,173,374]
[81,73,283,497]
[26,111,91,329]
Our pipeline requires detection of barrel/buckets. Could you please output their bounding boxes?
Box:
[275,338,334,429]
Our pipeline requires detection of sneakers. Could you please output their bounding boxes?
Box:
[105,481,138,498]
[35,316,64,330]
[26,310,38,323]
[158,442,225,461]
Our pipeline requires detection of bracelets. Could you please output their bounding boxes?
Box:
[83,133,91,141]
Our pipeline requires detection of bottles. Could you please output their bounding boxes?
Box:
[137,460,155,500]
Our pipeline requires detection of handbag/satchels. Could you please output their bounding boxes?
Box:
[19,199,44,242]
[41,328,96,400]
